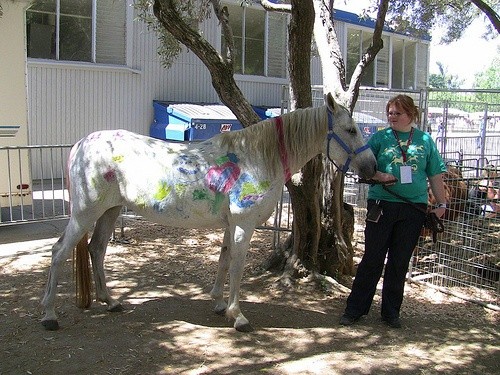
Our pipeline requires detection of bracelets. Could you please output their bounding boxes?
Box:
[438,203,446,208]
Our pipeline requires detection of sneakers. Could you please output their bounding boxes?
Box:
[380,315,401,328]
[339,313,359,325]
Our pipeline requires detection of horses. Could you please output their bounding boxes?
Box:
[414,161,500,287]
[40,92,378,332]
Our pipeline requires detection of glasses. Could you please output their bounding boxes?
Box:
[387,110,407,118]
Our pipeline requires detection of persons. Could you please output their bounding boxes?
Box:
[339,95,447,328]
[468,165,500,217]
